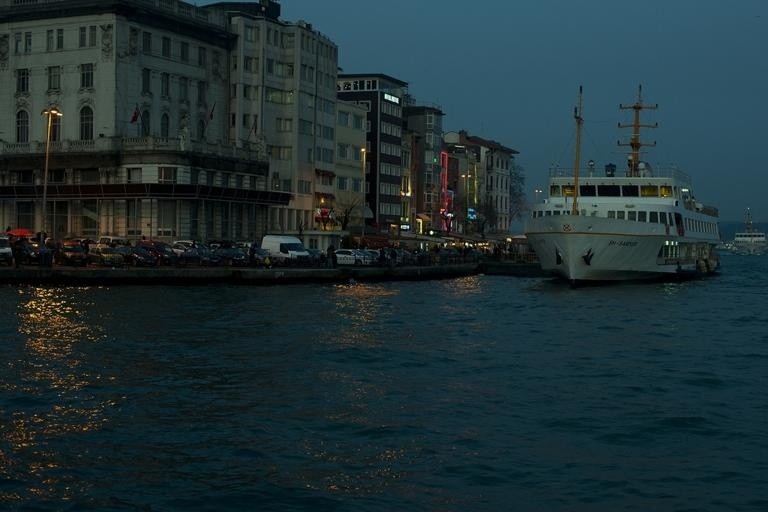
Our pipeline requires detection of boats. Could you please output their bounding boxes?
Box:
[524,81,724,287]
[729,206,767,256]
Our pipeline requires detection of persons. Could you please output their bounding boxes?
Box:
[377,244,440,265]
[318,244,338,270]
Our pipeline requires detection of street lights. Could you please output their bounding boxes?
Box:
[360,148,366,235]
[461,173,472,234]
[533,185,543,205]
[40,106,63,251]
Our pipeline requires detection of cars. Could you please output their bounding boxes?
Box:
[0,234,460,266]
